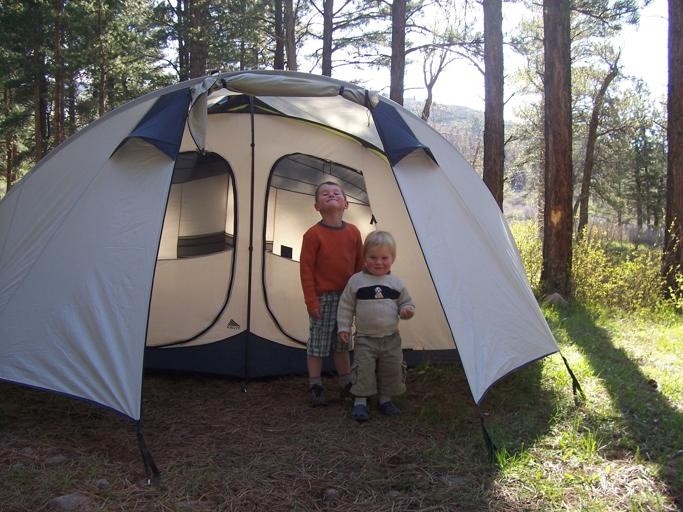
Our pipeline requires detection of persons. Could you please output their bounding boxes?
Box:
[300,180,415,422]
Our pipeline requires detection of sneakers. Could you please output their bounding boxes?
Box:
[378,401,400,416]
[341,382,354,401]
[351,404,368,421]
[310,384,327,407]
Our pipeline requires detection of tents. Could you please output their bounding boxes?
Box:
[0,72,585,480]
